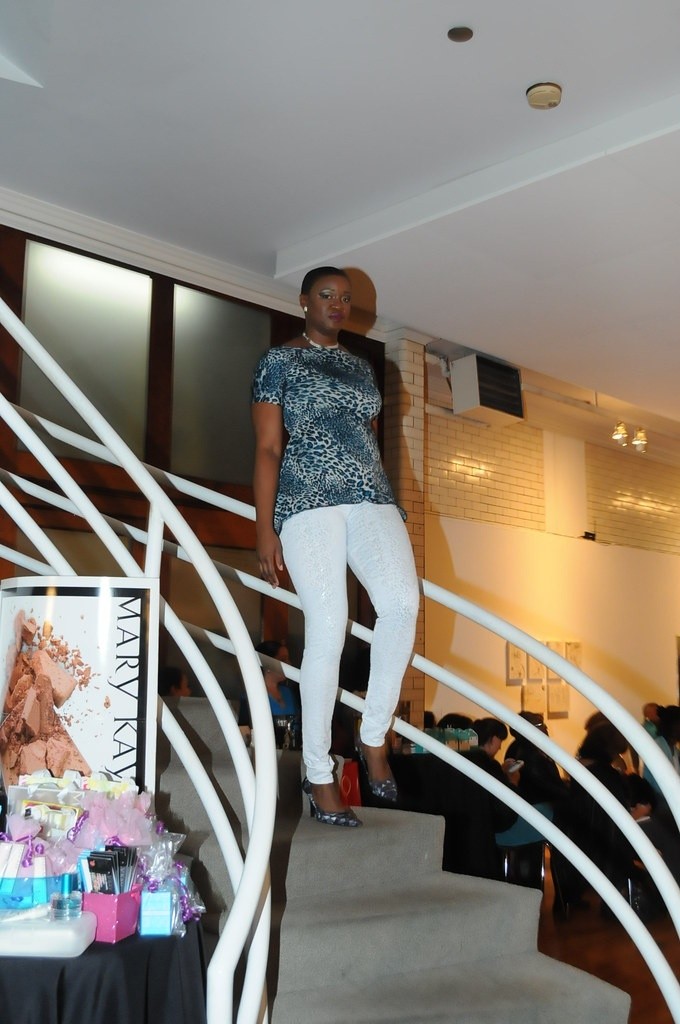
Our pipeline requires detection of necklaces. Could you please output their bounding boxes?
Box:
[302,330,341,350]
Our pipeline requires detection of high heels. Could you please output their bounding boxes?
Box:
[301,776,364,829]
[353,733,399,805]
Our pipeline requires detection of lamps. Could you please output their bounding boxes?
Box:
[632,427,648,453]
[612,421,629,446]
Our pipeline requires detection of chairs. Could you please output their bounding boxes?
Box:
[495,802,569,920]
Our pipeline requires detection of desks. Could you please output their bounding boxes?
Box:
[361,751,469,874]
[0,919,207,1024]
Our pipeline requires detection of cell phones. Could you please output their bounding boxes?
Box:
[509,760,524,773]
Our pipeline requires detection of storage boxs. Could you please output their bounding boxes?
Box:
[139,890,174,936]
[0,843,13,884]
[21,799,85,826]
[0,909,97,957]
[83,884,142,943]
[32,855,58,903]
[0,845,33,897]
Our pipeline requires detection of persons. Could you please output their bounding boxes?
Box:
[158,641,678,911]
[251,264,422,830]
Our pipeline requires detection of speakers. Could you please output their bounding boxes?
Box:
[450,354,524,428]
